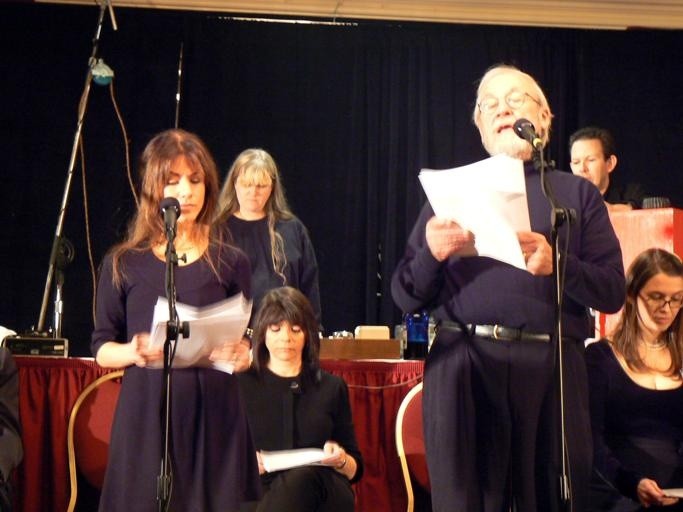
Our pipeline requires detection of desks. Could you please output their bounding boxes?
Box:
[13,355,425,512]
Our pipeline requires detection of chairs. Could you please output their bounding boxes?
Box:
[68,371,124,512]
[395,381,431,512]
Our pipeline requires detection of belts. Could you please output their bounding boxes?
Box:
[436,318,553,343]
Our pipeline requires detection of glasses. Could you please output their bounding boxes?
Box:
[473,91,544,113]
[627,292,683,312]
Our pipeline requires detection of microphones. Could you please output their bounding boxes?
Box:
[158,196,180,240]
[514,119,541,148]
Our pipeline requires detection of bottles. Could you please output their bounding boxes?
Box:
[401,310,429,360]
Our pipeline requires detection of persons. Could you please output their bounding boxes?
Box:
[239,283,363,512]
[0,325,25,511]
[389,62,624,512]
[93,129,262,512]
[561,122,643,214]
[583,247,683,512]
[211,141,323,351]
